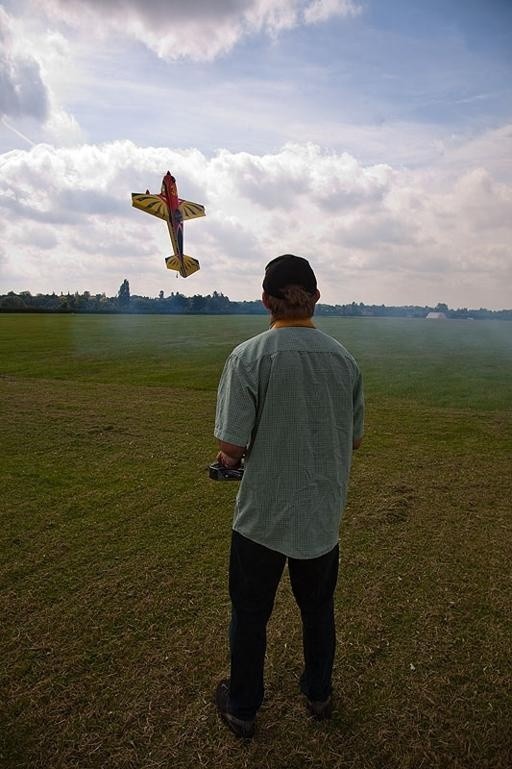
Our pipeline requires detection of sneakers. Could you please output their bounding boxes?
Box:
[215,676,258,740]
[300,689,337,719]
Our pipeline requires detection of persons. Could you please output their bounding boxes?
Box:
[209,254,370,737]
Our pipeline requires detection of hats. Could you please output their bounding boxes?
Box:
[261,253,317,298]
